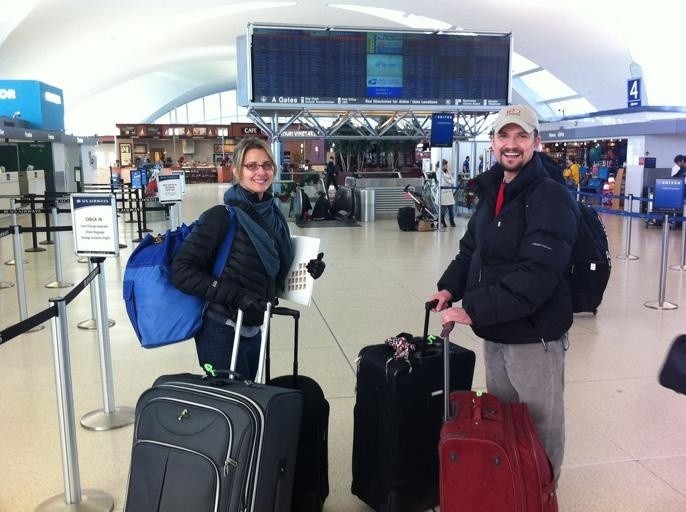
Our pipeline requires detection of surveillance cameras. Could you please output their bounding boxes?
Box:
[16,111,21,117]
[558,107,561,111]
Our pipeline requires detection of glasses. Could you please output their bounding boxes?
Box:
[241,161,273,172]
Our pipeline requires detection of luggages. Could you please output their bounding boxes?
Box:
[398,207,415,231]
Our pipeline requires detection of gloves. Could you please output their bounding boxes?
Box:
[306,252,326,279]
[235,289,268,314]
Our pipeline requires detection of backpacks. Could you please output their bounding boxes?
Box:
[476,175,611,313]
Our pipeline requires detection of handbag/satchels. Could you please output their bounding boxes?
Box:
[123,204,238,348]
[416,219,434,231]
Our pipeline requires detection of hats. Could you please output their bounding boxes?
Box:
[493,104,539,135]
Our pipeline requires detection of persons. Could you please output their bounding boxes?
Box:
[435,158,457,228]
[325,156,339,192]
[427,102,581,491]
[669,154,686,226]
[567,156,580,186]
[414,192,434,222]
[478,154,483,174]
[169,135,325,383]
[462,156,470,172]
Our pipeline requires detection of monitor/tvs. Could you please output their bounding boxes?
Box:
[431,113,453,147]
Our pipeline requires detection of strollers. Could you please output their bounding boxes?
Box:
[402,178,447,231]
